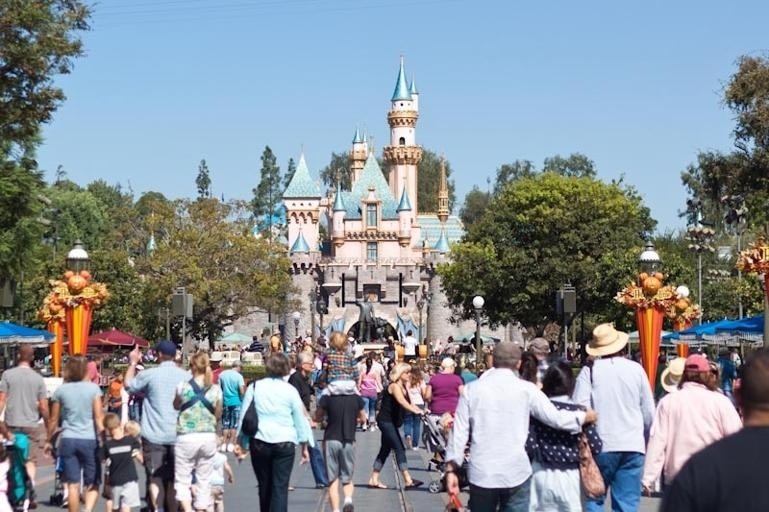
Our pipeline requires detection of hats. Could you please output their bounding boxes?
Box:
[152,341,176,355]
[660,358,685,392]
[686,354,709,372]
[585,324,628,356]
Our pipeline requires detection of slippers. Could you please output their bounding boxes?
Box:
[367,483,387,489]
[405,479,424,490]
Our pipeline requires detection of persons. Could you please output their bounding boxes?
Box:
[353,290,378,344]
[0,322,767,512]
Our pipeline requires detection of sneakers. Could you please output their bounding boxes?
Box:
[362,424,378,432]
[218,443,235,452]
[343,502,353,512]
[406,435,411,448]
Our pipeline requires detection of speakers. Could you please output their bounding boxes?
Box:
[556,290,563,314]
[563,287,578,313]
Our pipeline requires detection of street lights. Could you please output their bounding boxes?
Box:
[67,238,89,276]
[637,236,660,276]
[292,311,301,339]
[684,208,717,326]
[471,295,486,372]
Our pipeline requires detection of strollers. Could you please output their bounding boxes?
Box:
[420,414,469,495]
[2,440,35,511]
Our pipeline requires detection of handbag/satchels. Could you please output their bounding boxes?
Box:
[579,434,606,497]
[242,401,258,436]
